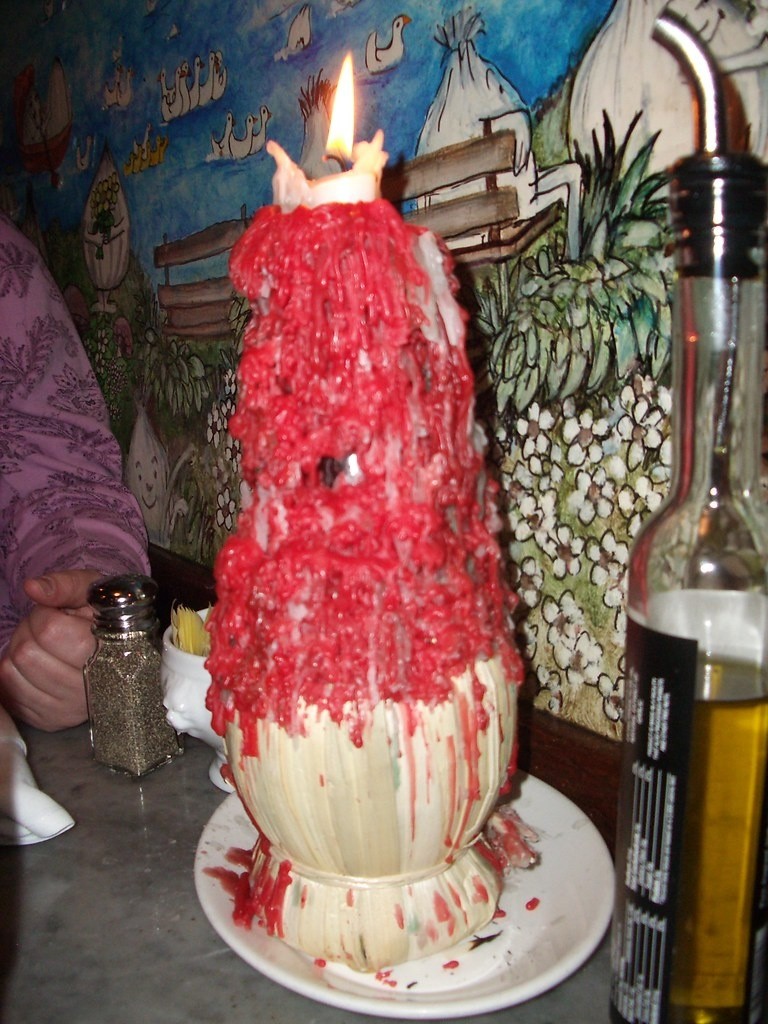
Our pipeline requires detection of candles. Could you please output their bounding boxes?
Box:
[268,48,389,215]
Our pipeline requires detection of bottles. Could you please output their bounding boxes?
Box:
[83,576,184,779]
[611,155,768,1024]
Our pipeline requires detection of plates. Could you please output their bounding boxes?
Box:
[194,772,617,1021]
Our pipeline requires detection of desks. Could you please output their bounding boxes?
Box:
[0,719,613,1024]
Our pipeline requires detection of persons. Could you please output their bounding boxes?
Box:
[0,213,151,731]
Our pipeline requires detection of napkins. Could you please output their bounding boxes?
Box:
[0,702,75,846]
